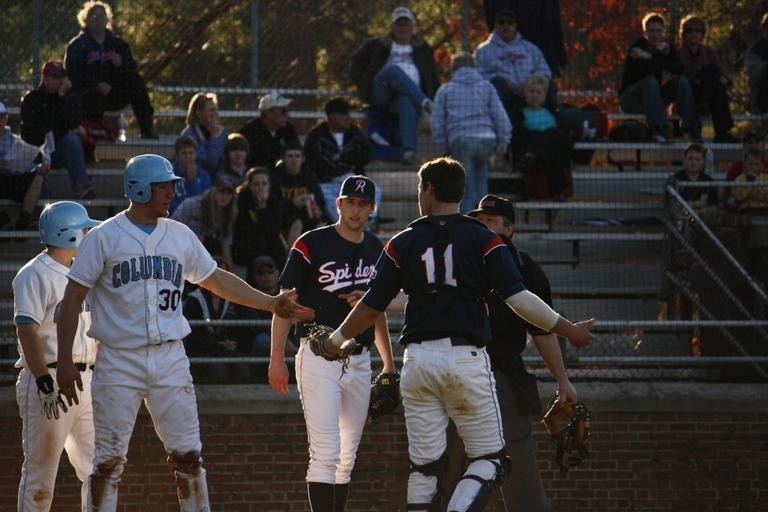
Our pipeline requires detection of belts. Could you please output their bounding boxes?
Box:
[450,335,472,346]
[352,346,363,355]
[46,362,94,371]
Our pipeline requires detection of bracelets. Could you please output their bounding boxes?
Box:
[329,327,346,347]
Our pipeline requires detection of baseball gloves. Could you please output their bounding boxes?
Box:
[368,370,400,419]
[540,391,592,471]
[304,322,357,362]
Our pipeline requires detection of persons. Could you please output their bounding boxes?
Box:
[473,7,552,81]
[268,176,396,512]
[339,194,579,511]
[664,131,768,227]
[619,6,768,143]
[56,154,316,512]
[165,91,381,381]
[348,6,437,162]
[431,51,513,212]
[0,1,160,223]
[319,156,597,510]
[504,73,569,201]
[12,200,104,511]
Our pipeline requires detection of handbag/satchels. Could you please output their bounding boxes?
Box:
[609,119,648,142]
[553,103,608,142]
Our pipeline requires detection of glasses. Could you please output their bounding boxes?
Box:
[497,20,515,25]
[685,28,701,33]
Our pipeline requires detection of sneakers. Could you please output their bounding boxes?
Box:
[519,156,532,172]
[649,129,739,144]
[0,212,13,231]
[141,122,159,139]
[14,214,38,231]
[403,148,417,163]
[75,191,96,200]
[553,194,565,202]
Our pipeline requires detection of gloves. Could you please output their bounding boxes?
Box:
[36,374,68,419]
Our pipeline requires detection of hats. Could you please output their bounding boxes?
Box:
[215,175,234,191]
[467,195,514,222]
[339,176,376,204]
[253,256,276,273]
[391,6,413,23]
[258,94,293,111]
[43,61,67,77]
[325,99,357,113]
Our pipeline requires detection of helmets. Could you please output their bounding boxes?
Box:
[123,154,185,203]
[38,201,102,248]
